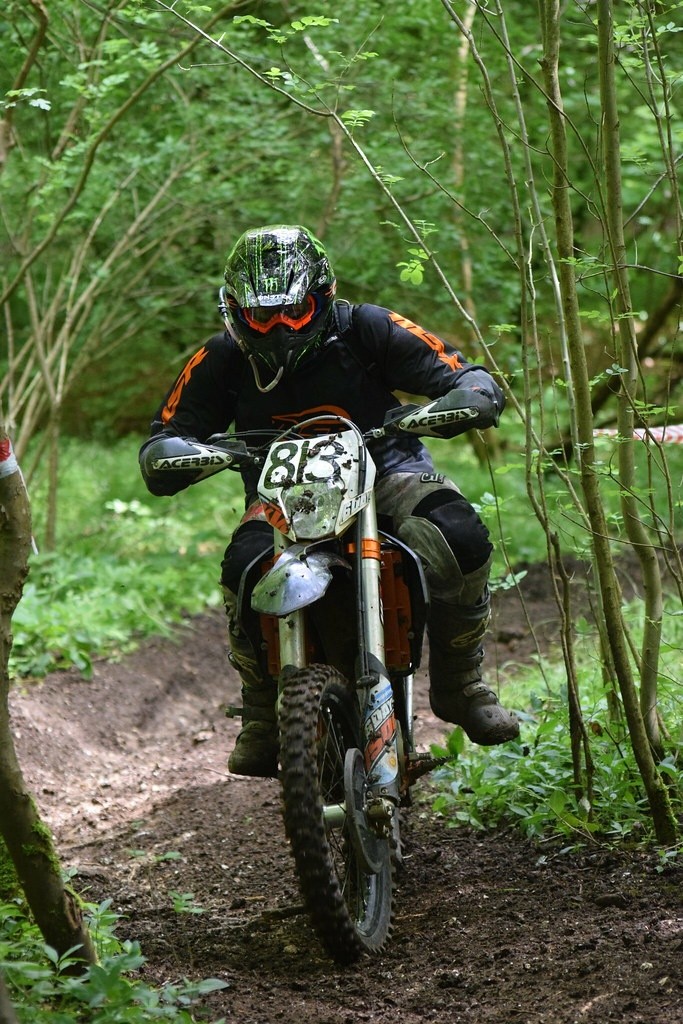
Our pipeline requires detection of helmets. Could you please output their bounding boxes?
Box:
[224,224,336,377]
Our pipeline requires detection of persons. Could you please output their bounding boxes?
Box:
[134,222,523,778]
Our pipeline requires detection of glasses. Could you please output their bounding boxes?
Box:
[243,298,314,333]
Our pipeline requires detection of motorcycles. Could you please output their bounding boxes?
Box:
[139,389,500,969]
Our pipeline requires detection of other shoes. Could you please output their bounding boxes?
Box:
[227,690,280,777]
[428,647,521,745]
